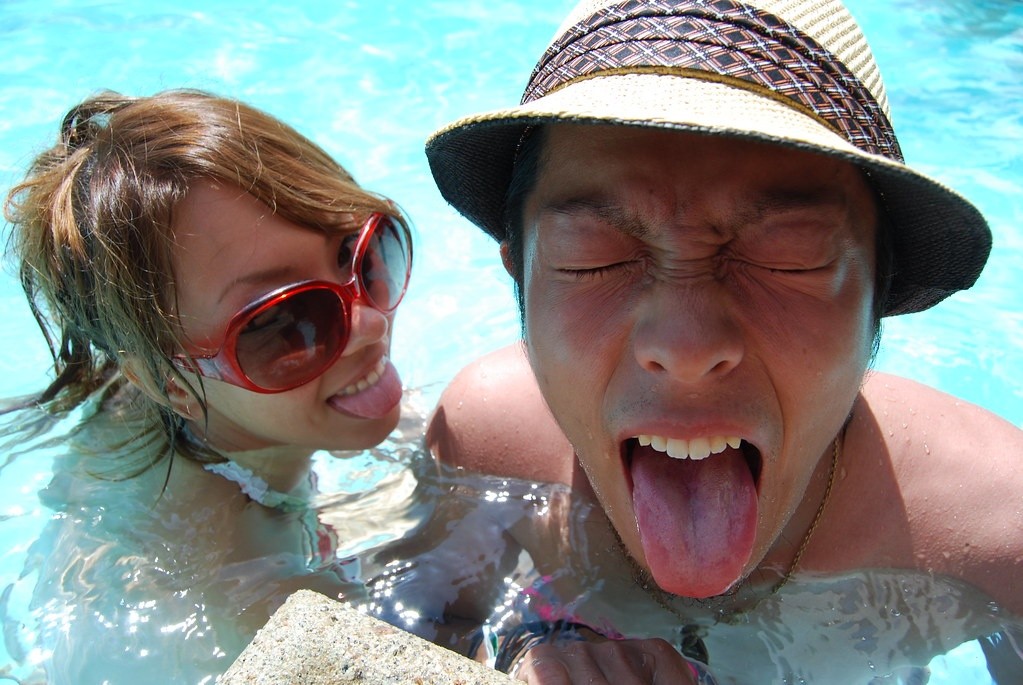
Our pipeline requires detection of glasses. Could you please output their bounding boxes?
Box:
[174,199,413,393]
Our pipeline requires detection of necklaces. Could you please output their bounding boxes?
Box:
[604,409,839,664]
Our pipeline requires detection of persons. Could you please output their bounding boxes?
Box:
[0,90,417,456]
[422,0,1023,685]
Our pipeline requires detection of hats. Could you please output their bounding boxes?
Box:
[424,1,994,318]
